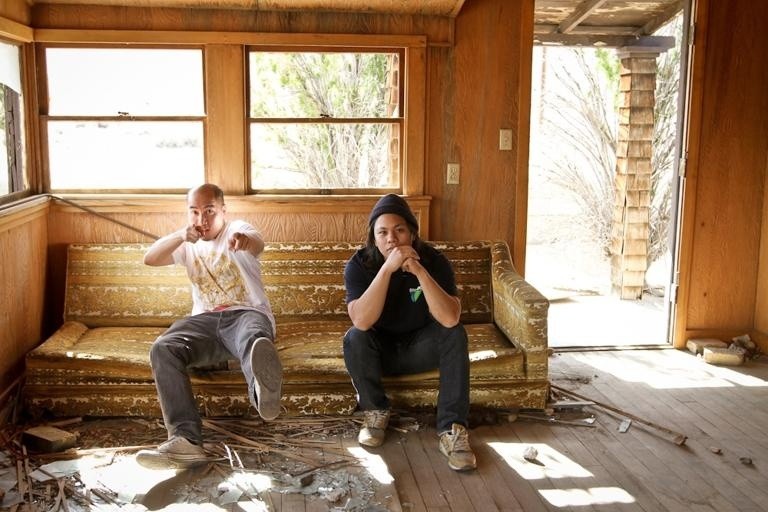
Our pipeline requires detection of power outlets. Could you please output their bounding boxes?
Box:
[446,164,460,184]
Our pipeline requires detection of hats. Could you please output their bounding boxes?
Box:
[368,194,418,229]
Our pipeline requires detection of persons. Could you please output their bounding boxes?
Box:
[343,193,477,473]
[136,183,284,470]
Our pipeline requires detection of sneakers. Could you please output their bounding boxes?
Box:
[439,423,477,471]
[136,436,208,471]
[357,409,391,447]
[251,337,283,421]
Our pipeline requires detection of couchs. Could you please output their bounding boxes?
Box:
[23,237,549,422]
